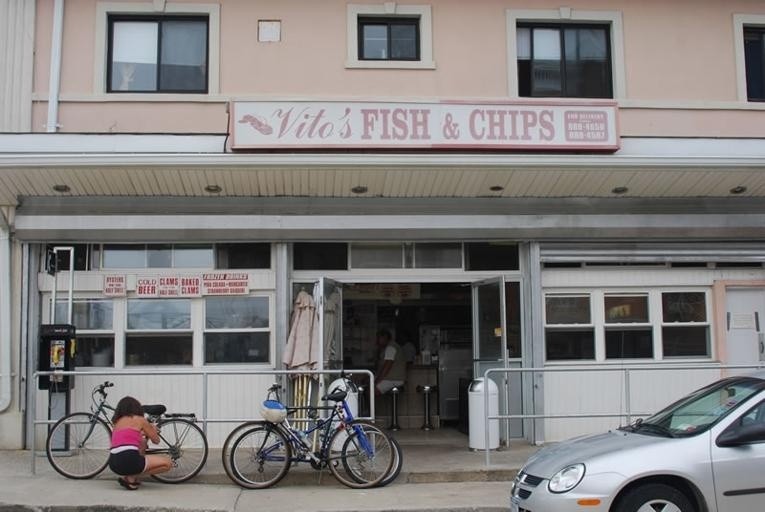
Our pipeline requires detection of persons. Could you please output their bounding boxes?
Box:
[375,330,408,396]
[108,396,171,490]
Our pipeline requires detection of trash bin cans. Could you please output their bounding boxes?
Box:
[327,377,358,452]
[467,377,500,452]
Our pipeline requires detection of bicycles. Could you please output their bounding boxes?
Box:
[44,380,208,484]
[219,372,404,490]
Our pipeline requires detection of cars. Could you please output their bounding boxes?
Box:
[509,369,764,511]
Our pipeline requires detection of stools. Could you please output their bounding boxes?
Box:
[387,385,403,431]
[356,384,369,415]
[416,384,437,431]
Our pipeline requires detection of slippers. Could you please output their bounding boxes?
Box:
[119,476,139,490]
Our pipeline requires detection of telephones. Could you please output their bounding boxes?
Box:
[50,340,65,368]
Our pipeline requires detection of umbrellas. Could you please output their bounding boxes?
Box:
[281,291,341,453]
